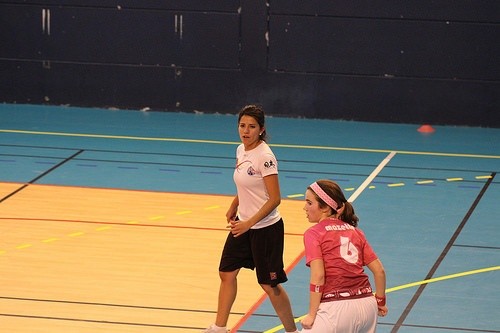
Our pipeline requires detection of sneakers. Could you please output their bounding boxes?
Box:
[205,325,227,333]
[285,329,298,333]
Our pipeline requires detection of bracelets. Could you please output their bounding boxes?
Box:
[310,282,325,293]
[298,179,388,333]
[375,294,386,306]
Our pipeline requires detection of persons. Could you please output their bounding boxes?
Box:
[202,104,300,333]
